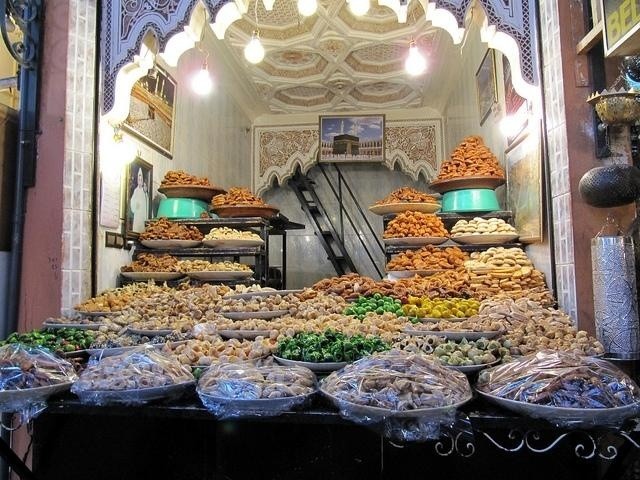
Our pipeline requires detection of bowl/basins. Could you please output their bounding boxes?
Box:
[156,197,208,219]
[443,188,500,214]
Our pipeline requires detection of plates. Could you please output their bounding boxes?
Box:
[127,327,169,338]
[42,323,102,332]
[219,329,270,339]
[157,185,221,199]
[405,329,500,338]
[226,309,286,319]
[211,206,278,218]
[74,382,184,403]
[392,269,441,277]
[0,382,73,404]
[225,291,284,300]
[85,346,133,359]
[429,176,507,192]
[202,239,264,249]
[319,387,473,415]
[157,342,185,349]
[184,271,251,280]
[452,234,518,243]
[369,203,442,214]
[446,356,502,371]
[270,352,348,373]
[383,237,448,246]
[76,312,107,317]
[64,349,88,357]
[194,389,315,408]
[475,386,640,424]
[121,271,182,280]
[421,317,467,324]
[140,239,200,248]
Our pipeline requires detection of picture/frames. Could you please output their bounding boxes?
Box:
[474,46,498,127]
[503,118,547,244]
[124,155,154,240]
[112,62,177,159]
[317,112,386,163]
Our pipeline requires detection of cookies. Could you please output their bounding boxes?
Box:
[450,217,516,234]
[465,247,546,291]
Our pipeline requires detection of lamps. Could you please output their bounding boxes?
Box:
[405,4,430,78]
[186,10,214,94]
[242,0,265,65]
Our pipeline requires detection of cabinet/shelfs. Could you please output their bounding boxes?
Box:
[0,208,640,462]
